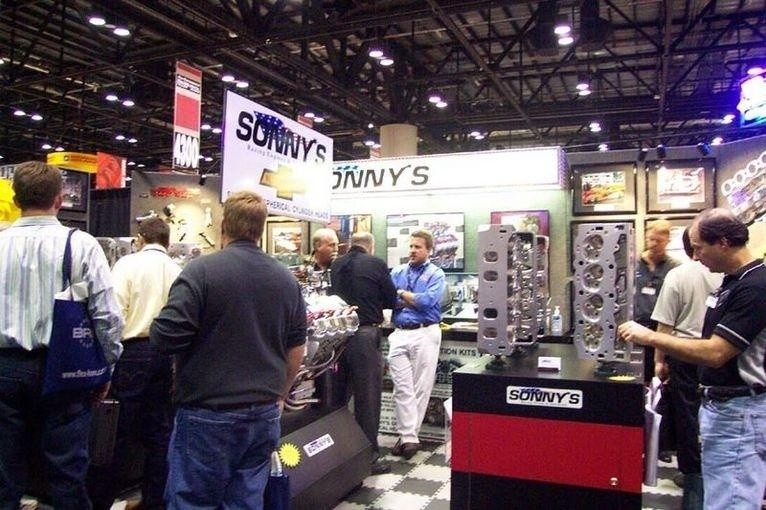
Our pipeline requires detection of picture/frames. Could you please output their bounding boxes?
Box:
[266,219,302,255]
[308,214,372,256]
[645,157,717,213]
[386,212,465,270]
[571,161,637,216]
[490,209,551,298]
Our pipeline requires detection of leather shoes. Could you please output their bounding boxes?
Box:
[401,444,421,461]
[371,460,392,475]
[391,440,404,457]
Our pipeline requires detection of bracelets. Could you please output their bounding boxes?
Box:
[397,289,405,298]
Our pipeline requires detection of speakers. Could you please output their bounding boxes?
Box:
[274,406,372,510]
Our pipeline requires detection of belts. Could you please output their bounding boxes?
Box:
[695,385,765,402]
[397,321,436,329]
[360,322,379,327]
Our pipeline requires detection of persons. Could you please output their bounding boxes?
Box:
[148,194,307,510]
[389,230,447,459]
[301,228,339,291]
[88,217,182,510]
[633,220,683,463]
[110,239,137,267]
[328,230,397,476]
[617,207,766,510]
[0,161,126,510]
[650,225,727,488]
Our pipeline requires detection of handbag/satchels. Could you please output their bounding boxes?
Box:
[40,228,113,404]
